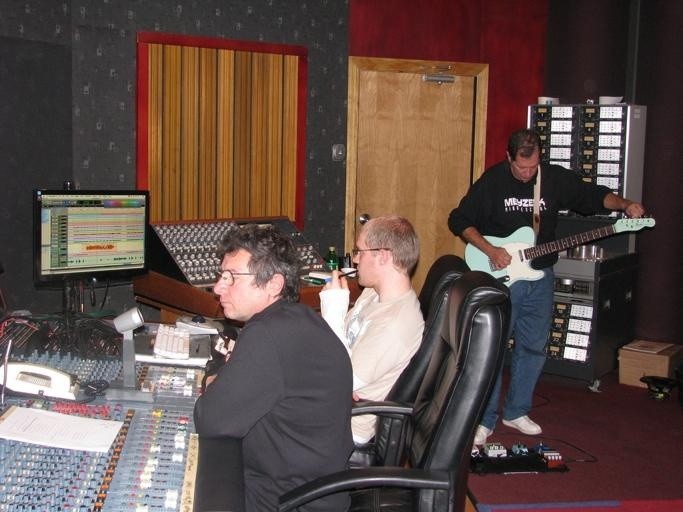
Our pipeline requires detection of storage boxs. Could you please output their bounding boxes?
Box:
[617,339,683,389]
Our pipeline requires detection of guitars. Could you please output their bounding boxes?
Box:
[464,215,657,288]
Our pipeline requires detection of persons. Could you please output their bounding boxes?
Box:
[317,214,425,445]
[447,129,646,447]
[192,220,357,510]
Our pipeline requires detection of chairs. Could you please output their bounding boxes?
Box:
[349,253,474,470]
[273,271,512,511]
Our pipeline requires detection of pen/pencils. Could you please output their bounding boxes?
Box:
[338,270,358,278]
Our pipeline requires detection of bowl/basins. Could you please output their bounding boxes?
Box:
[568,245,607,260]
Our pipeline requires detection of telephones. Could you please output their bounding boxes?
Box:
[0,362,81,401]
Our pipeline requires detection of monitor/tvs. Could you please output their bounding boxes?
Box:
[33,189,149,286]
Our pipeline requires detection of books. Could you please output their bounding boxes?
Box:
[306,269,332,280]
[133,334,211,369]
[297,274,324,285]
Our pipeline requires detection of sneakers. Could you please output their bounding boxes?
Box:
[498,414,544,436]
[472,423,494,446]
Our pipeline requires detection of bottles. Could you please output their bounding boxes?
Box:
[324,246,339,271]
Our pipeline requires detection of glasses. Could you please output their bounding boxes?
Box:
[351,246,394,259]
[213,270,259,286]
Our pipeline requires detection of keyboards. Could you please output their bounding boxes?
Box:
[153,323,190,360]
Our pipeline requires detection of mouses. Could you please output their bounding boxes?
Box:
[175,314,220,335]
